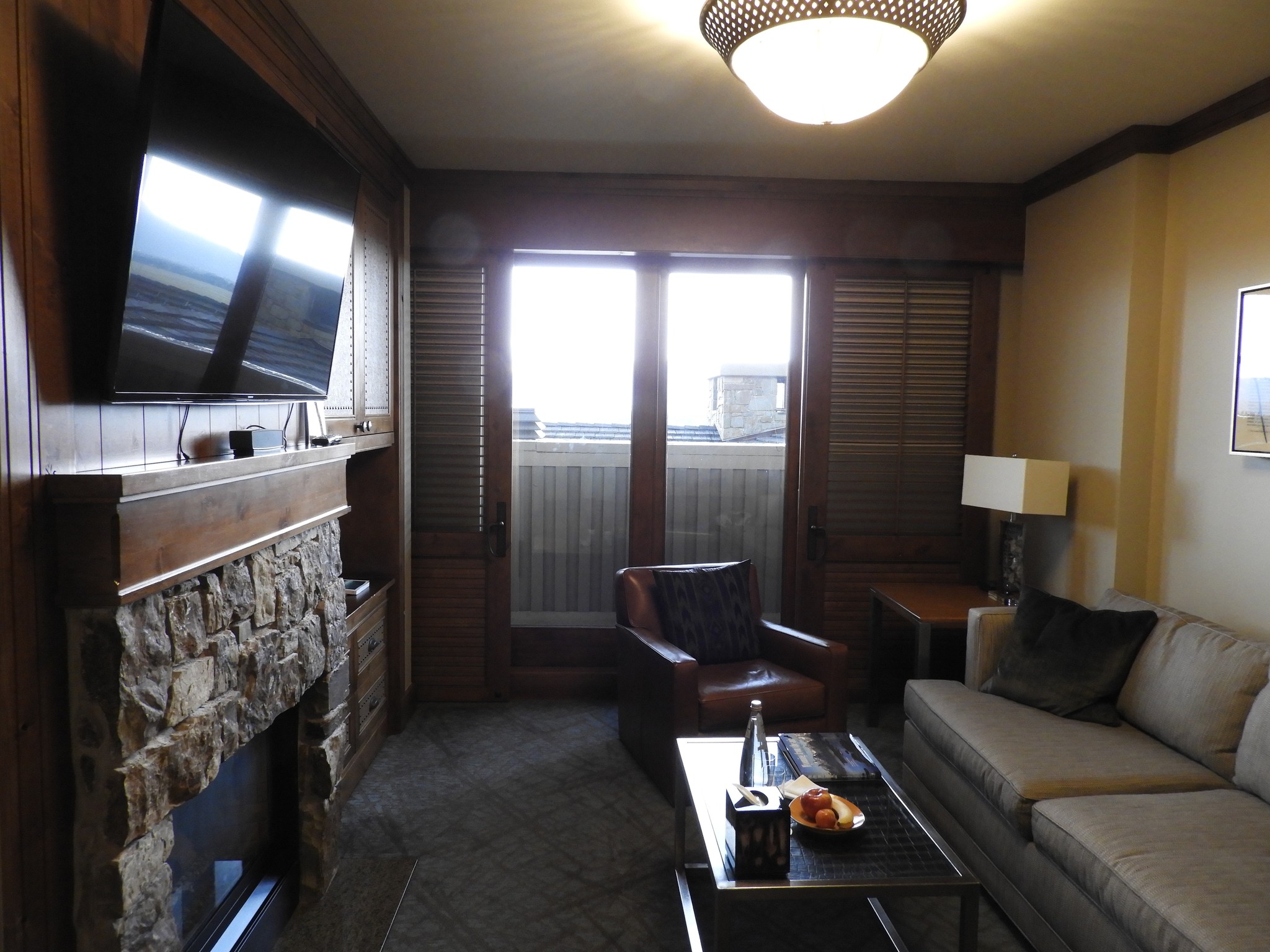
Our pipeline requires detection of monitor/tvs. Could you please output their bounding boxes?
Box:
[101,0,361,403]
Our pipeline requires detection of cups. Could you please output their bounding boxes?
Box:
[761,765,785,787]
[752,752,775,787]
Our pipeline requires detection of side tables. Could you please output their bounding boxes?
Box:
[859,574,1003,733]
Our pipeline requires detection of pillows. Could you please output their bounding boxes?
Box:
[980,581,1158,729]
[653,558,765,665]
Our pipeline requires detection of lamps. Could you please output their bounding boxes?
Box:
[698,0,970,126]
[959,452,1070,605]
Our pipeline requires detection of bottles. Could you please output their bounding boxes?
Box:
[739,700,768,788]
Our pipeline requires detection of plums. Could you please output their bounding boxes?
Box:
[815,809,836,828]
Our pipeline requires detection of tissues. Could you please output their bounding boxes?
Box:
[724,785,792,877]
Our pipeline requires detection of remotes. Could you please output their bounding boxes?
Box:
[311,435,342,446]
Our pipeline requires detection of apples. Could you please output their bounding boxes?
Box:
[800,788,833,820]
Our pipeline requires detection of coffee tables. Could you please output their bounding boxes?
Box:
[670,731,984,952]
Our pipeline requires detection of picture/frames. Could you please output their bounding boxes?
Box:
[1225,279,1270,461]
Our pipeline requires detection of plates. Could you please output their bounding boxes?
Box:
[789,793,866,836]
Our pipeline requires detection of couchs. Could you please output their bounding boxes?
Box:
[900,585,1270,952]
[611,557,852,812]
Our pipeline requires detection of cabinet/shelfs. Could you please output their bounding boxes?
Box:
[343,574,395,805]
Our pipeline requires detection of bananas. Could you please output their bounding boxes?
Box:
[831,797,854,828]
